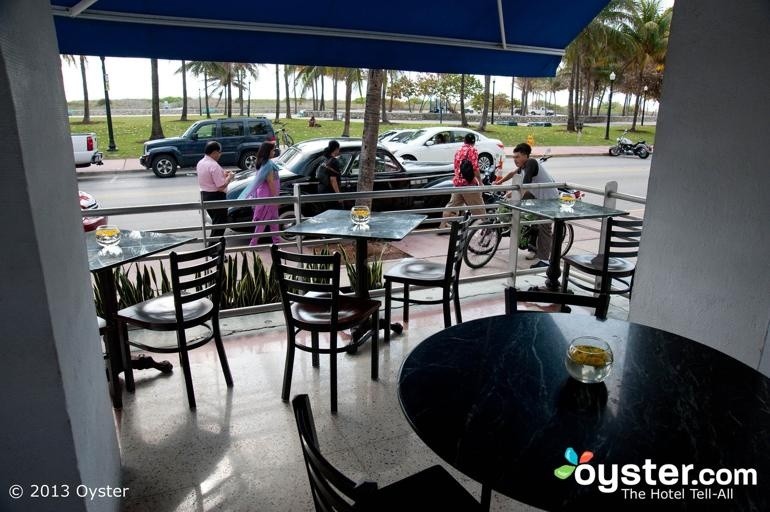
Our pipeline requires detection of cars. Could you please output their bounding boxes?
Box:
[79,191,108,231]
[381,129,430,145]
[388,127,505,173]
[378,130,402,142]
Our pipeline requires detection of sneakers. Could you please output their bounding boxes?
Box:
[525,251,536,259]
[530,261,551,269]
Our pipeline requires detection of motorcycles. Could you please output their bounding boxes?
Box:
[608,129,653,159]
[537,148,585,202]
[465,159,513,220]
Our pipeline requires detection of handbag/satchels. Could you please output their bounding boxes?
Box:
[460,150,475,184]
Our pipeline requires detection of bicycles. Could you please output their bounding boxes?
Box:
[465,182,566,254]
[464,193,574,269]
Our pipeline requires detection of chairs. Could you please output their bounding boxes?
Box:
[112,236,234,410]
[383,208,472,343]
[292,394,481,512]
[562,215,642,297]
[504,285,611,318]
[270,245,382,414]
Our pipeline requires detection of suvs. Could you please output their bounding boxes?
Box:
[139,116,281,178]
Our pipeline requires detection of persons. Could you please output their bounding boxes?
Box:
[503,145,560,268]
[239,141,290,247]
[435,134,445,144]
[494,143,538,262]
[439,133,486,235]
[316,141,346,211]
[195,142,236,262]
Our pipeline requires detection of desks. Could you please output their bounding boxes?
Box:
[83,227,198,389]
[282,209,429,355]
[396,310,770,512]
[499,196,630,302]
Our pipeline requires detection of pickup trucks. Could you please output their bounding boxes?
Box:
[530,107,554,115]
[71,133,104,167]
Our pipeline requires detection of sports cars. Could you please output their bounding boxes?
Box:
[199,137,465,243]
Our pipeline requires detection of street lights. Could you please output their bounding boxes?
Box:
[641,84,649,127]
[604,71,616,139]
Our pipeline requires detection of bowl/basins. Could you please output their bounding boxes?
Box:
[558,193,578,209]
[97,224,123,244]
[348,206,376,225]
[569,338,615,381]
